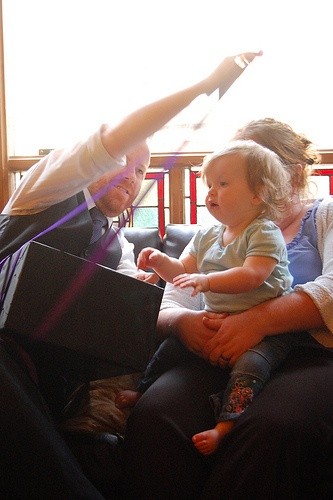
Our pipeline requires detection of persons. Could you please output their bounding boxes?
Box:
[125,115,333,500]
[115,140,303,458]
[0,49,268,500]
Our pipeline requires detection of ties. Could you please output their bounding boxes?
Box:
[89,209,108,246]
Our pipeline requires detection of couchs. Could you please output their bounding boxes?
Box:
[157,223,204,290]
[116,226,161,289]
